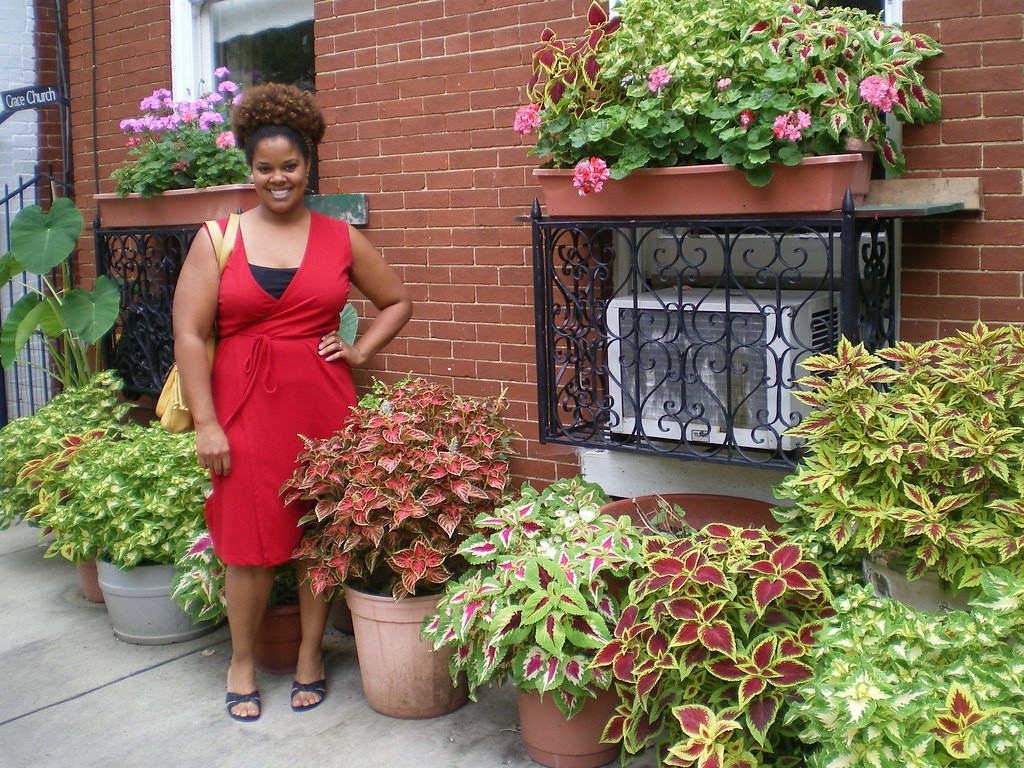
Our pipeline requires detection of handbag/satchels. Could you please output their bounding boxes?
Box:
[156,327,215,434]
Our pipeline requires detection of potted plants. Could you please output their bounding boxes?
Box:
[1,320,1024,767]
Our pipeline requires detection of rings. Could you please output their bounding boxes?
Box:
[339,342,342,350]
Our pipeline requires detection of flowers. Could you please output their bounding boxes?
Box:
[513,1,942,197]
[108,65,252,198]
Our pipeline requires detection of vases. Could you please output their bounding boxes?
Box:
[532,153,862,217]
[844,136,877,194]
[94,184,262,226]
[596,492,791,605]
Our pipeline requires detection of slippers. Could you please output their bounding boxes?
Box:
[291,679,327,712]
[226,689,261,722]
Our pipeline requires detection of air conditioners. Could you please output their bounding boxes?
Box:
[608,284,844,451]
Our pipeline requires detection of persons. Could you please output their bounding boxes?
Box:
[173,84,415,721]
